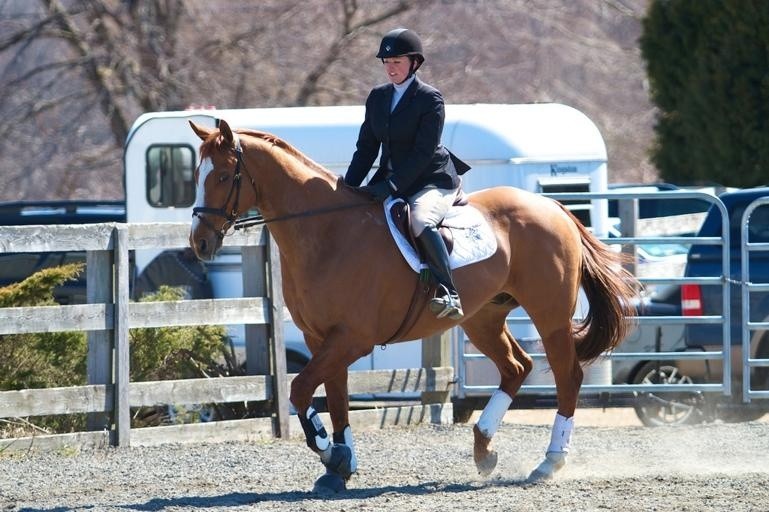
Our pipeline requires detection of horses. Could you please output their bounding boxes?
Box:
[182,118,649,497]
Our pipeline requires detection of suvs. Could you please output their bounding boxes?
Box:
[606,222,719,429]
[608,177,748,247]
[0,194,121,311]
[680,183,769,412]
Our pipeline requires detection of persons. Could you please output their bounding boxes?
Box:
[345,27,473,319]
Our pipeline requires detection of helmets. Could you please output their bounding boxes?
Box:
[374,26,427,62]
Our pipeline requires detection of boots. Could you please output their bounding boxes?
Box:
[414,221,465,322]
